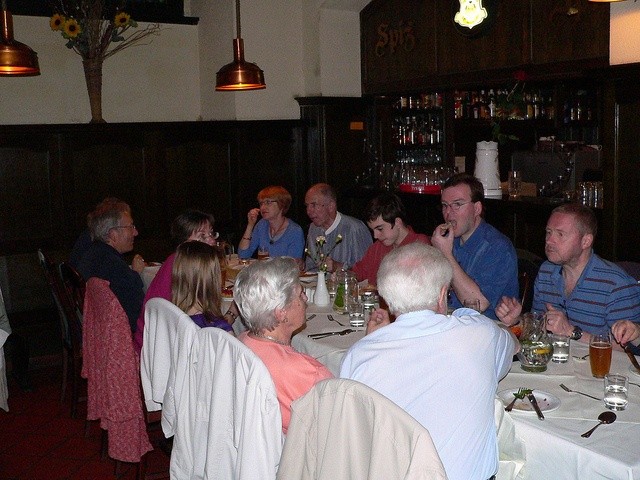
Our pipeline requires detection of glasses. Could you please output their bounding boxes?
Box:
[108,225,136,230]
[259,200,278,206]
[440,200,472,212]
[190,231,219,242]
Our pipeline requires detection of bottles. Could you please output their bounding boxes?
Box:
[568,97,600,143]
[393,116,443,146]
[456,89,548,122]
[392,150,459,185]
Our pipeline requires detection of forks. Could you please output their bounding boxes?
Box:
[506,387,527,411]
[328,314,345,328]
[559,383,602,403]
[305,314,316,321]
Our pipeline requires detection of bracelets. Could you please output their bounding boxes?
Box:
[241,236,251,240]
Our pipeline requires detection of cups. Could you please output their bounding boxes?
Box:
[588,333,612,379]
[464,298,481,313]
[348,301,365,327]
[603,376,626,409]
[552,336,570,363]
[507,169,525,200]
[360,290,380,313]
[225,244,270,267]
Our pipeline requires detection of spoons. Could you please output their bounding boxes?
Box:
[582,411,617,440]
[313,328,352,339]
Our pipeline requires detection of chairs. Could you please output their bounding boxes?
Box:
[84,280,143,474]
[60,259,88,460]
[163,328,280,476]
[35,247,89,421]
[140,300,197,430]
[278,376,449,480]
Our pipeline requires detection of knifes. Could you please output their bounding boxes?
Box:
[525,390,545,421]
[307,329,364,337]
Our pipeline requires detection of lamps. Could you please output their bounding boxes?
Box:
[1,0,42,76]
[214,1,265,94]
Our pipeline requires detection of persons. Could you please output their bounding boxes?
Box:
[130,203,241,366]
[349,191,433,322]
[237,184,305,260]
[232,256,335,436]
[431,172,520,321]
[495,201,640,351]
[612,319,640,346]
[305,183,373,273]
[63,226,145,277]
[79,197,147,338]
[170,240,238,338]
[339,242,514,480]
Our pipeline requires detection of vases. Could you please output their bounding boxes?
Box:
[81,59,108,125]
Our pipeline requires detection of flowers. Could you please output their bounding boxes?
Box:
[48,1,163,58]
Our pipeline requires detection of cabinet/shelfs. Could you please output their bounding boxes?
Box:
[366,90,601,213]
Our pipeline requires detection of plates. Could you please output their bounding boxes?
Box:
[499,387,561,414]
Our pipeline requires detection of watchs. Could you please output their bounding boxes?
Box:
[571,326,583,340]
